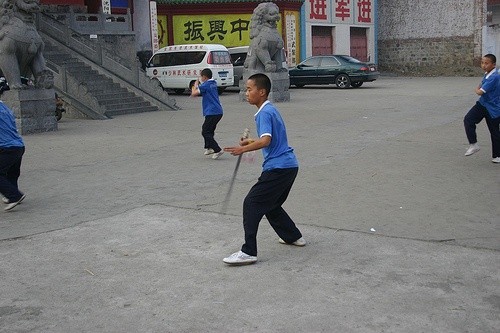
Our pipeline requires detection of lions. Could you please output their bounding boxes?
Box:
[0,0,54,89]
[244,2,287,72]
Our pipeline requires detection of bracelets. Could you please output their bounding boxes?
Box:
[248,138,251,144]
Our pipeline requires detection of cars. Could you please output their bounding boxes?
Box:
[288,54,379,89]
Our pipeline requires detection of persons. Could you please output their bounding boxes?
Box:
[223,74,306,263]
[463,54,500,163]
[192,68,224,160]
[0,101,27,210]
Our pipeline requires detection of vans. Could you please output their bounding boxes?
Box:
[145,43,235,96]
[222,45,249,91]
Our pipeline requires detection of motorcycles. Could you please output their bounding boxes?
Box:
[55,96,63,121]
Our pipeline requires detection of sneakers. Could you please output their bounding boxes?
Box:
[2,192,26,210]
[464,142,480,156]
[492,156,500,163]
[222,249,258,265]
[212,150,224,159]
[278,237,306,246]
[204,148,214,155]
